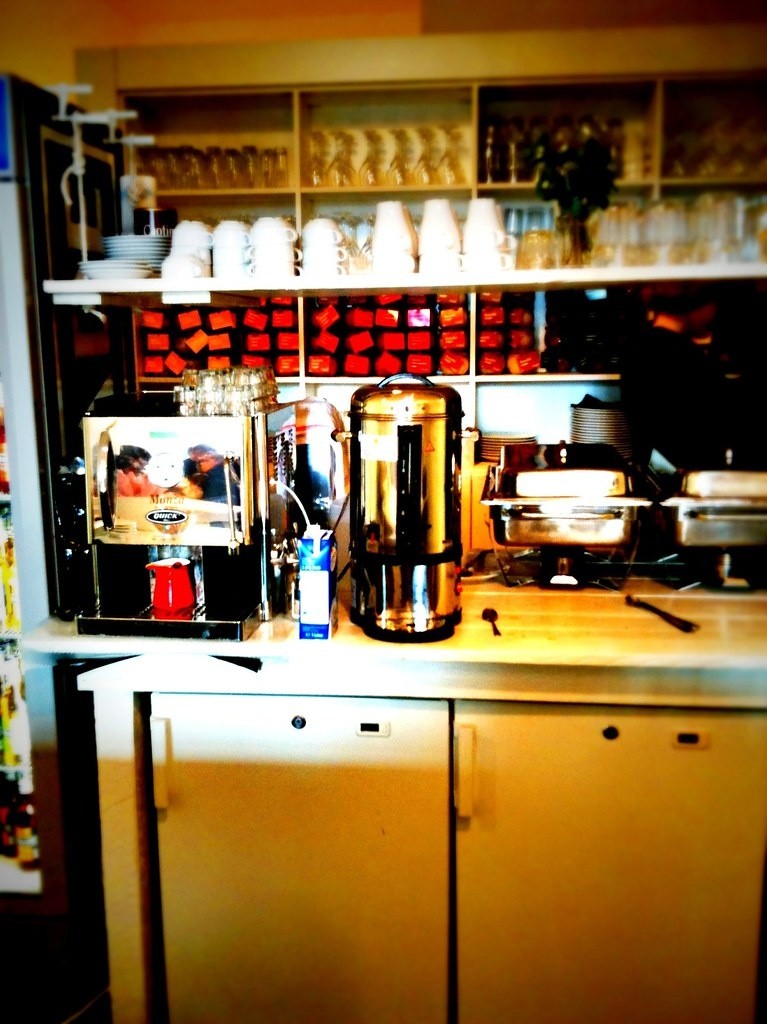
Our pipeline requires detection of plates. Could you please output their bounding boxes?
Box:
[77,232,170,278]
[480,430,537,462]
[570,405,625,446]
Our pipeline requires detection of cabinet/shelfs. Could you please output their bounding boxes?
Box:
[118,69,766,385]
[146,695,767,1024]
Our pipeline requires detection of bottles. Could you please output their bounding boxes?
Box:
[138,141,289,189]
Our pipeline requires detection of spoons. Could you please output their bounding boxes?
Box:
[480,605,502,636]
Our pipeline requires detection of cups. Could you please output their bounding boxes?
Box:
[462,198,507,271]
[589,191,749,269]
[176,365,279,418]
[145,558,196,620]
[518,229,569,266]
[162,218,302,280]
[417,198,460,272]
[370,200,416,271]
[302,215,349,280]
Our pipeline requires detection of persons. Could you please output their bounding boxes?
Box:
[107,443,242,507]
